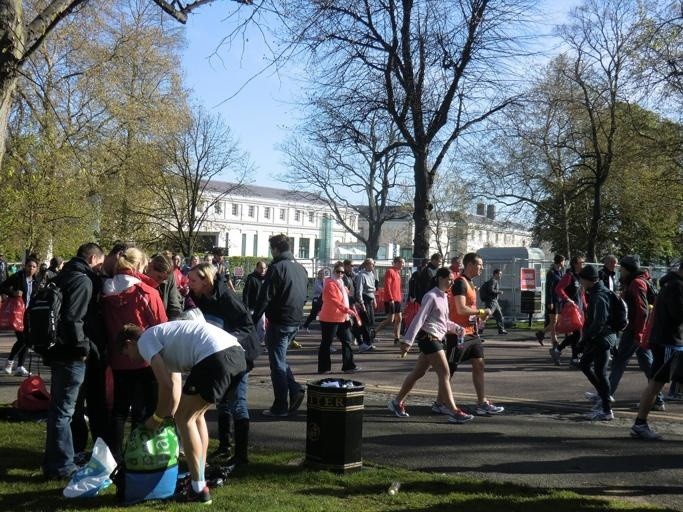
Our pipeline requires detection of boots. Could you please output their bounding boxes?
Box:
[206,413,232,464]
[220,417,248,467]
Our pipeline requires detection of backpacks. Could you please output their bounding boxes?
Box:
[480,278,498,302]
[23,278,66,357]
[590,289,630,330]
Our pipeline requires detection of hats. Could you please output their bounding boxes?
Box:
[579,263,599,281]
[619,255,640,274]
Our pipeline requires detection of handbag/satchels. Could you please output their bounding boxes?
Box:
[12,376,50,411]
[109,414,178,506]
[0,294,25,331]
[404,302,421,329]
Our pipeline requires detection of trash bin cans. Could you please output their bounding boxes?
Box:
[306,377,365,475]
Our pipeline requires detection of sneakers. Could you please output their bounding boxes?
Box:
[73,449,91,466]
[14,366,32,377]
[288,390,304,414]
[42,464,79,481]
[173,474,210,503]
[358,342,371,353]
[4,360,14,374]
[536,330,683,440]
[387,398,503,422]
[498,329,508,334]
[262,409,289,417]
[290,341,302,349]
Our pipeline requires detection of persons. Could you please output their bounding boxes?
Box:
[251,234,309,416]
[408,252,462,307]
[477,268,508,335]
[242,262,268,346]
[536,253,683,443]
[3,241,259,481]
[117,320,248,504]
[342,258,378,350]
[387,267,475,423]
[432,253,505,416]
[303,269,326,331]
[316,261,362,375]
[375,256,407,345]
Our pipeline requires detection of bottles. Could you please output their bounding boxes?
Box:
[456,326,465,351]
[387,480,401,497]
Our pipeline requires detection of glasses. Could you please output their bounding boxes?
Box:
[334,270,346,274]
